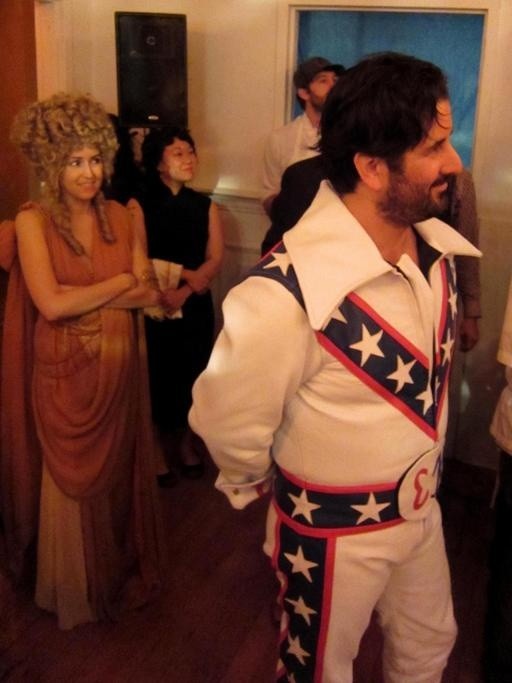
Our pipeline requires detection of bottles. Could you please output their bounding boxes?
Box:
[292,55,344,87]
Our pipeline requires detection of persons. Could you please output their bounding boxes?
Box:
[126,125,225,490]
[258,56,345,221]
[478,280,511,681]
[12,89,165,632]
[448,169,482,356]
[189,50,482,681]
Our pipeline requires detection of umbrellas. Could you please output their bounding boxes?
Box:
[157,470,177,488]
[178,458,203,481]
